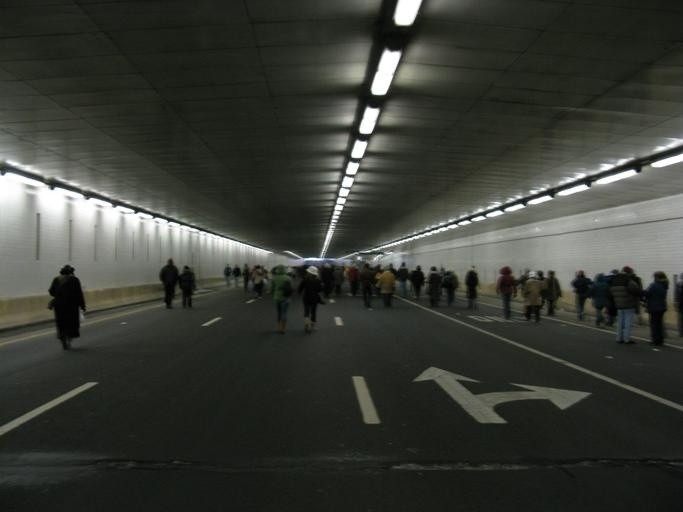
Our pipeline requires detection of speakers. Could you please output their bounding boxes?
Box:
[305,318,315,332]
[276,320,286,333]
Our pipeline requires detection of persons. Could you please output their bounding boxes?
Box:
[242,262,276,298]
[296,266,322,331]
[642,271,669,345]
[496,266,517,320]
[515,270,562,323]
[158,259,180,309]
[571,266,644,345]
[287,264,360,300]
[358,263,378,309]
[178,265,197,309]
[224,263,231,289]
[427,266,459,307]
[232,264,241,287]
[465,270,479,310]
[46,262,87,355]
[269,264,295,333]
[374,262,425,308]
[673,283,682,336]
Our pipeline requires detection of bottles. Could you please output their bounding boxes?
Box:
[60,265,74,275]
[307,266,319,275]
[501,267,511,274]
[528,270,539,278]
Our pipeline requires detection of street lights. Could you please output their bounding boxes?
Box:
[48,299,56,310]
